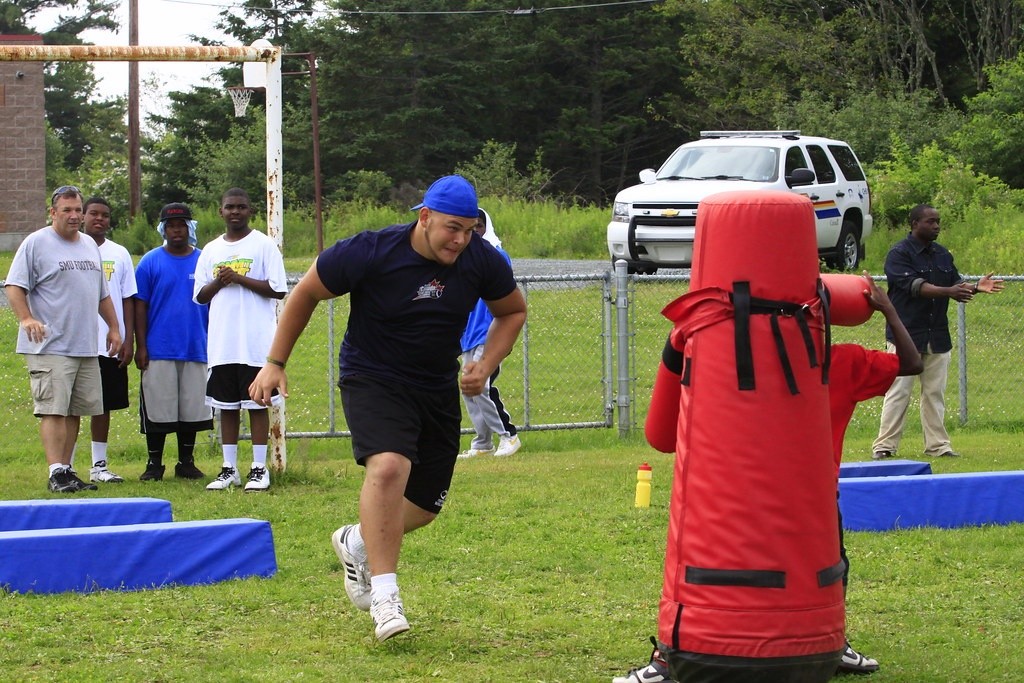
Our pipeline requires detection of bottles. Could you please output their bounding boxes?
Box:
[635,463,652,508]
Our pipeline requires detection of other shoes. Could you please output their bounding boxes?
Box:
[873,450,894,461]
[939,451,961,458]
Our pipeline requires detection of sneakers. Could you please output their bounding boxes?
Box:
[839,645,879,671]
[367,589,410,642]
[332,524,371,612]
[492,434,522,458]
[47,467,75,493]
[244,461,271,493]
[612,650,672,683]
[205,461,241,490]
[456,445,497,460]
[139,458,166,483]
[90,462,124,484]
[174,463,207,482]
[63,468,99,491]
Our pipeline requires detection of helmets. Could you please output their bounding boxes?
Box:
[159,202,192,222]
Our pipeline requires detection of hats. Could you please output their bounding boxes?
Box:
[410,173,480,219]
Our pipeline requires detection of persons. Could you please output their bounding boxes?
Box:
[134,202,215,481]
[455,207,522,459]
[71,196,139,484]
[5,186,124,494]
[613,268,923,683]
[873,204,1005,460]
[193,187,289,488]
[248,174,527,644]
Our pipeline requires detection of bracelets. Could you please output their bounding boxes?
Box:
[974,281,978,293]
[267,358,287,369]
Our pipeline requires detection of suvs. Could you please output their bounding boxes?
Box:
[602,129,877,270]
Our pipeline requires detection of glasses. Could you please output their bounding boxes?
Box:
[51,186,81,206]
